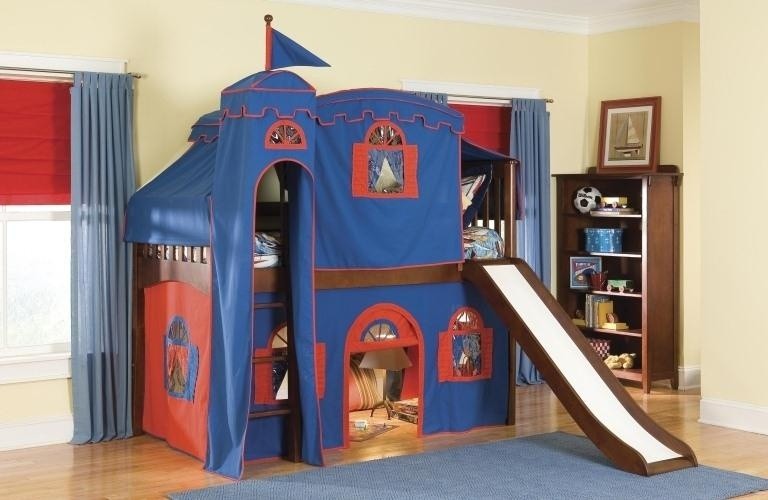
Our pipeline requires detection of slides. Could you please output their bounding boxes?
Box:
[466,258,700,475]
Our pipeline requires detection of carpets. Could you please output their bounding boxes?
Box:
[166,429,768,499]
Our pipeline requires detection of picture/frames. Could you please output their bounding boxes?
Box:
[596,96,662,175]
[568,256,603,290]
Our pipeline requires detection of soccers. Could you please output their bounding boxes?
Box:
[573,186,602,215]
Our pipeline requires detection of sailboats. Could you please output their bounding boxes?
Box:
[611,116,641,154]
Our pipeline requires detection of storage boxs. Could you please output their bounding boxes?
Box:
[584,227,624,253]
[588,338,611,362]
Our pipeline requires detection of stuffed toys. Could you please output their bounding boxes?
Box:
[603,352,638,370]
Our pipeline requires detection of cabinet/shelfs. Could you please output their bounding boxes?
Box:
[550,162,684,394]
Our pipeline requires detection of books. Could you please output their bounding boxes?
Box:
[572,292,630,330]
